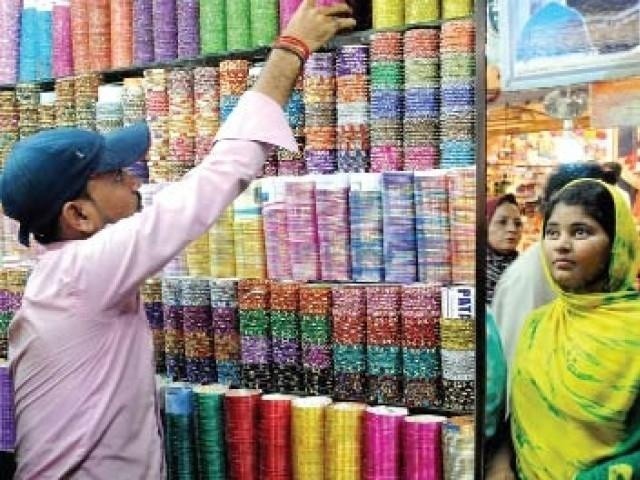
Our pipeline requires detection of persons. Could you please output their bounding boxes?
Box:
[478,158,640,480]
[0,0,359,480]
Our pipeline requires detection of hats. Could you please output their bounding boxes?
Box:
[1,121,149,245]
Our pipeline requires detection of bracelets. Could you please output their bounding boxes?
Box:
[270,32,312,65]
